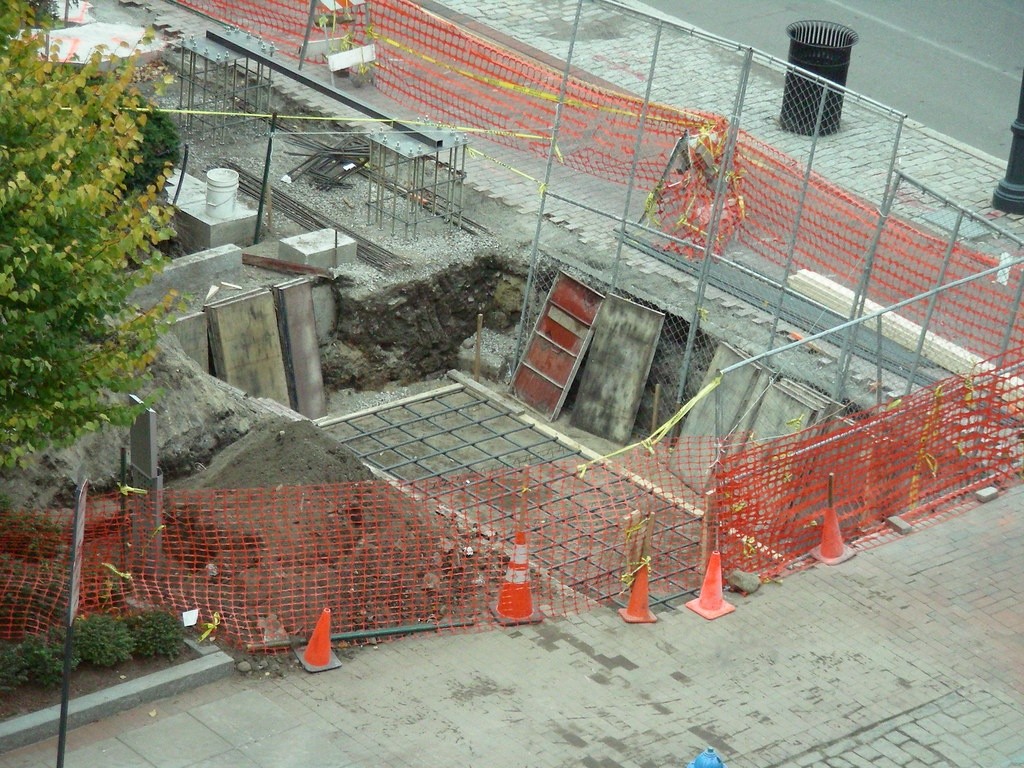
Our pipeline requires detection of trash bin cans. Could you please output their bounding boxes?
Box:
[781,19,859,137]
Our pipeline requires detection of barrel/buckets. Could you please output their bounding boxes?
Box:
[203,168,239,218]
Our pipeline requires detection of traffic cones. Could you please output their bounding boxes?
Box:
[683,549,738,620]
[810,508,857,568]
[290,609,342,674]
[487,530,545,628]
[617,556,657,624]
